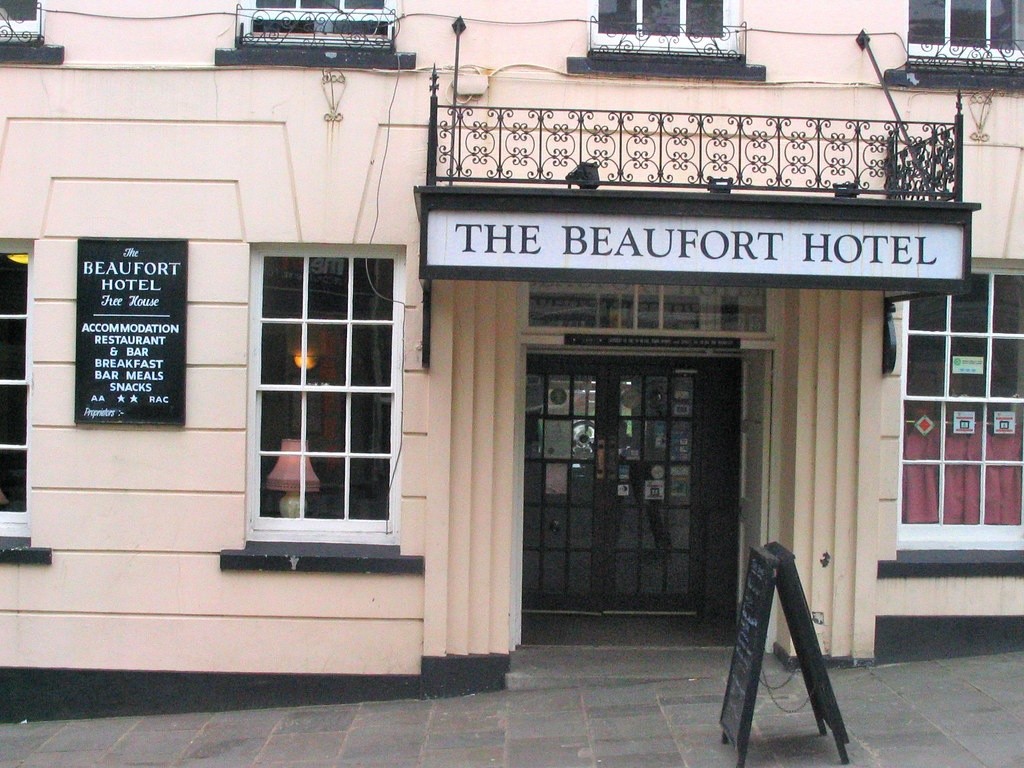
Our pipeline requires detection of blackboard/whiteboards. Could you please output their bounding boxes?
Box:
[719,543,781,755]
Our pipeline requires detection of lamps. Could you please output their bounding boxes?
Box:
[566,162,600,189]
[266,439,320,518]
